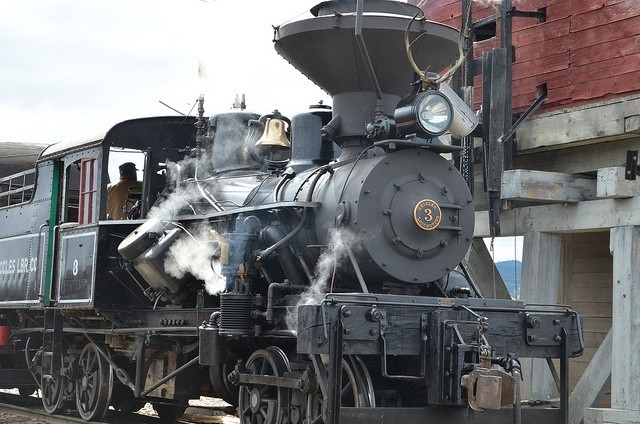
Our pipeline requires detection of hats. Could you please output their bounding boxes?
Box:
[119,163,138,174]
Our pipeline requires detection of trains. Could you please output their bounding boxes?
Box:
[0,0,584,424]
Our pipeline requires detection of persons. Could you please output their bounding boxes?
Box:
[105,160,146,219]
